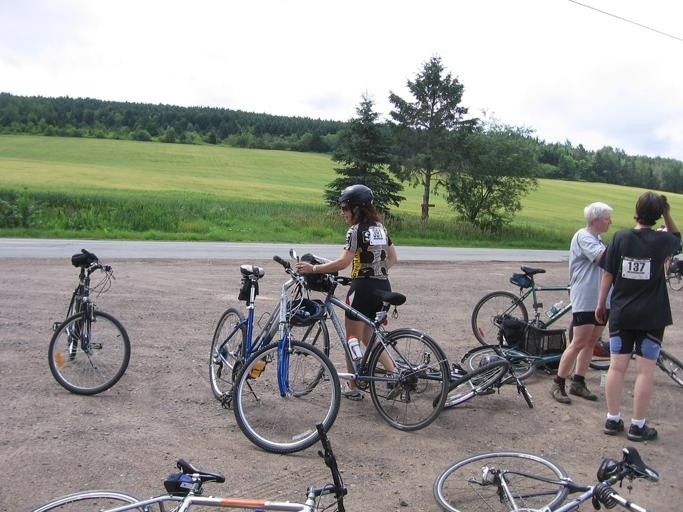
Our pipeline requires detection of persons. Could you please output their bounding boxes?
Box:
[655,225,665,232]
[296,185,398,400]
[593,193,682,442]
[549,202,615,404]
[663,256,671,282]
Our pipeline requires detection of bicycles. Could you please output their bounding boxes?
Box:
[394,263,682,409]
[665,250,682,292]
[47,248,132,396]
[288,248,453,432]
[207,255,343,454]
[30,420,347,511]
[434,446,663,511]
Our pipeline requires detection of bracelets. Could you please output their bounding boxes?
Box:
[310,263,317,276]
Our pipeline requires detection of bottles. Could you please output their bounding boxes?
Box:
[544,301,563,317]
[346,330,363,364]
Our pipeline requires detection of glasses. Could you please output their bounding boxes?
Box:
[339,205,350,211]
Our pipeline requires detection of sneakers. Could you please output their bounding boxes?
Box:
[627,425,658,442]
[387,375,398,388]
[340,382,363,400]
[604,419,624,434]
[548,381,571,403]
[569,379,598,401]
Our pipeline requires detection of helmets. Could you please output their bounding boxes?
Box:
[287,298,322,326]
[338,184,374,205]
